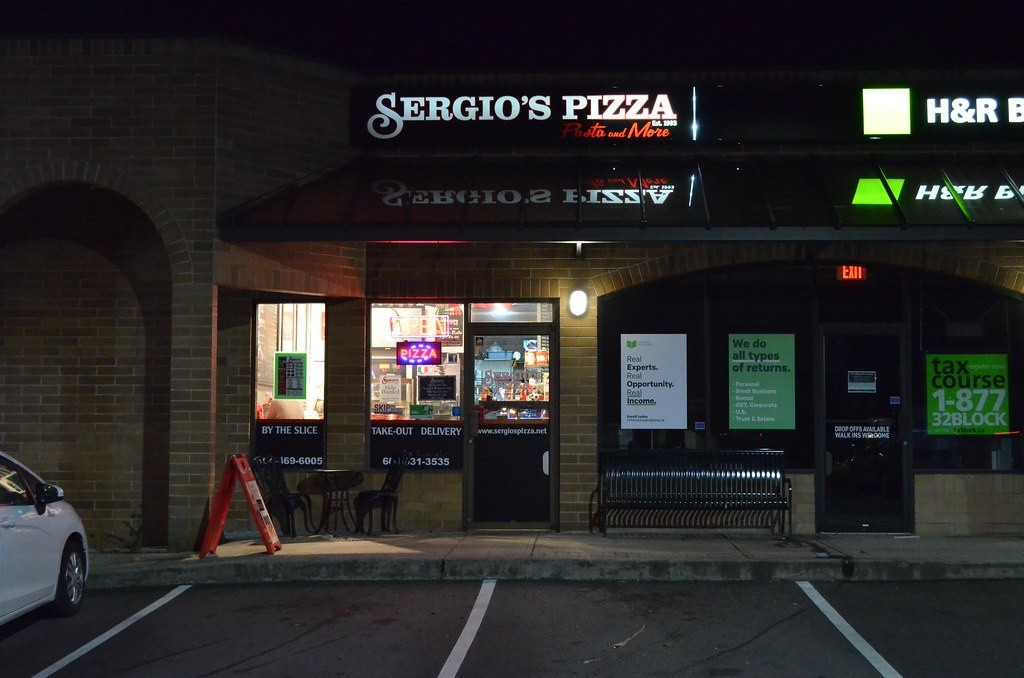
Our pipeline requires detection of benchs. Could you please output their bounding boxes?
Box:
[598,450,793,540]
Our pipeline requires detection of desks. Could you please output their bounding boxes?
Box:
[302,468,364,534]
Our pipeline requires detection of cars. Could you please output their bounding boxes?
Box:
[0,453,91,626]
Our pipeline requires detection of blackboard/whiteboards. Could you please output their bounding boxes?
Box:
[417,375,457,401]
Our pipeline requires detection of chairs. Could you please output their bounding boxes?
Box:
[254,454,315,536]
[355,448,411,534]
